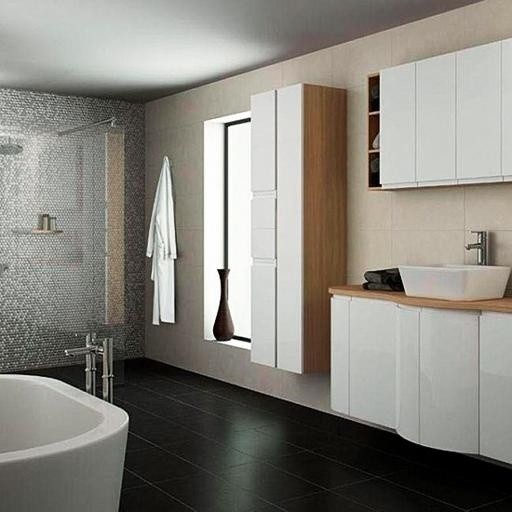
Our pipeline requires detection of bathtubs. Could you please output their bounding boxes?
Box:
[0,373,130,512]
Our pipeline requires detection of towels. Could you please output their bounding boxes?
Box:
[363,267,403,291]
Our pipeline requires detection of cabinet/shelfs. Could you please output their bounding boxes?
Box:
[479,306,511,465]
[395,296,479,461]
[364,37,512,190]
[249,82,347,375]
[327,287,396,431]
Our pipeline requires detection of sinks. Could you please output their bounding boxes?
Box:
[397,263,512,301]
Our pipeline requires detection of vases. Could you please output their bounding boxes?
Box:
[214,268,235,342]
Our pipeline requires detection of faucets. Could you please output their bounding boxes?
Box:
[64,330,114,403]
[464,229,489,266]
